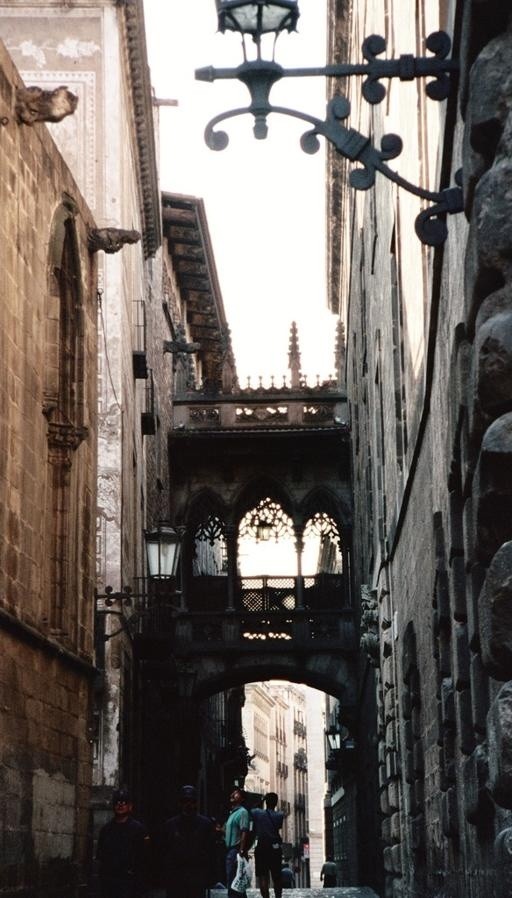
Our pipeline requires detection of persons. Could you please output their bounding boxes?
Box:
[213,784,250,898]
[152,784,227,898]
[249,791,285,898]
[96,787,151,898]
[281,862,296,888]
[320,854,338,888]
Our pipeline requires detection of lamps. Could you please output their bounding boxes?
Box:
[195,0,465,250]
[92,518,187,652]
[325,725,341,751]
